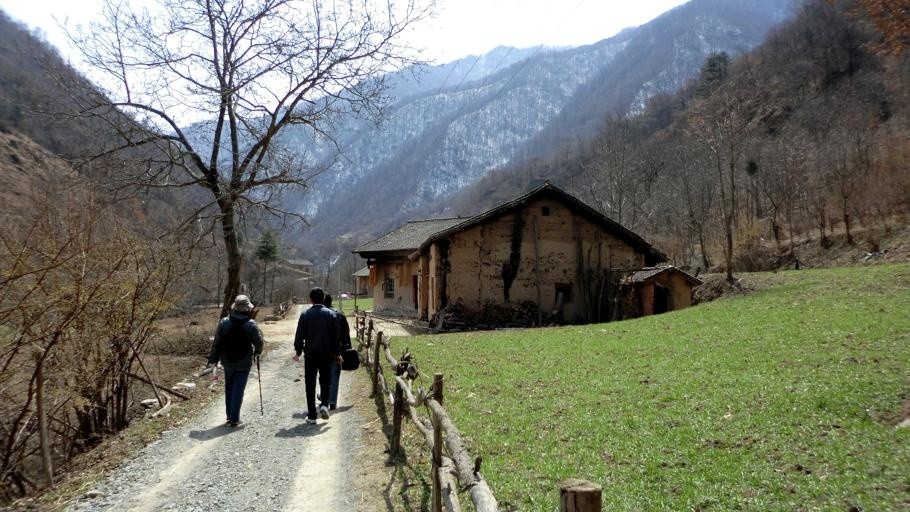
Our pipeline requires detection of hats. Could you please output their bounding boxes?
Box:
[230,294,255,314]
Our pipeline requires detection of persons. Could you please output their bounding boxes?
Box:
[316,295,351,409]
[293,287,343,425]
[207,295,263,426]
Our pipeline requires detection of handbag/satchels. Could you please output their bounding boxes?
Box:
[339,344,359,370]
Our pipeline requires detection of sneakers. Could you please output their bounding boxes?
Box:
[223,418,243,427]
[305,416,315,425]
[320,406,330,420]
[317,394,336,410]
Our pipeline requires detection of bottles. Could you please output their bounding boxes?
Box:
[211,362,220,387]
